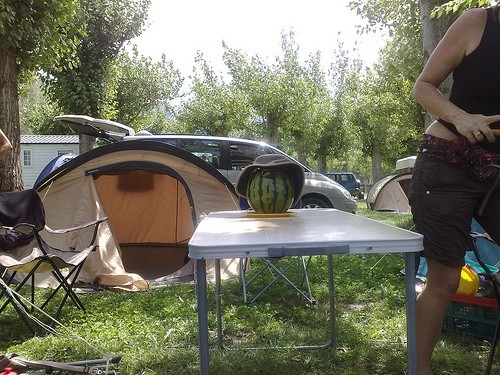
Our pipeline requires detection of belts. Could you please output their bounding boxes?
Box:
[417,133,500,186]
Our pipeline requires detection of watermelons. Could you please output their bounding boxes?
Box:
[246,168,294,214]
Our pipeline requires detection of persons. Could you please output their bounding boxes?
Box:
[0,129,12,155]
[407,1,500,375]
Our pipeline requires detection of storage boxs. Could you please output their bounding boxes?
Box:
[442,295,499,346]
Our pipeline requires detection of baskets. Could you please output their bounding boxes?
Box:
[442,282,500,340]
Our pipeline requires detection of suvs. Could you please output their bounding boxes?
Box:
[326,172,361,196]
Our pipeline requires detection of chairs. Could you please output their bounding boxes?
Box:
[0,190,109,335]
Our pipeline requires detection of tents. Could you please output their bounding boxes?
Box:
[6,139,250,292]
[366,172,414,213]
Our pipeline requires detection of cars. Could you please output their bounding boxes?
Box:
[32,115,358,215]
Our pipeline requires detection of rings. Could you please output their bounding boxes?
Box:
[474,132,481,137]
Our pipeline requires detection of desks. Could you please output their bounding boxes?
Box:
[188,208,424,375]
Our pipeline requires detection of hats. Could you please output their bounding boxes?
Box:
[238,149,304,209]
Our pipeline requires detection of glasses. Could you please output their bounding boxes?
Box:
[253,167,294,180]
